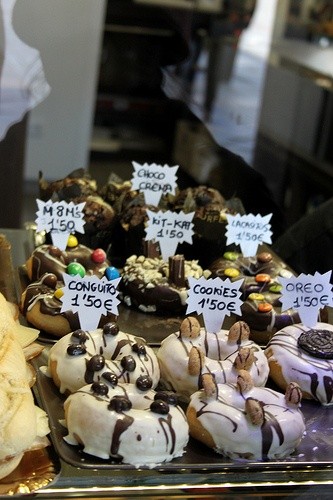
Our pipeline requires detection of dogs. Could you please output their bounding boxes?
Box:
[174,99,277,220]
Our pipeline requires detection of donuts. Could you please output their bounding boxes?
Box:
[207,249,294,301]
[116,254,212,317]
[235,284,303,343]
[39,321,161,395]
[263,322,333,404]
[18,235,120,290]
[154,316,271,398]
[187,371,308,460]
[19,273,120,338]
[57,369,190,466]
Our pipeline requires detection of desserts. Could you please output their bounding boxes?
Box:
[35,167,242,256]
